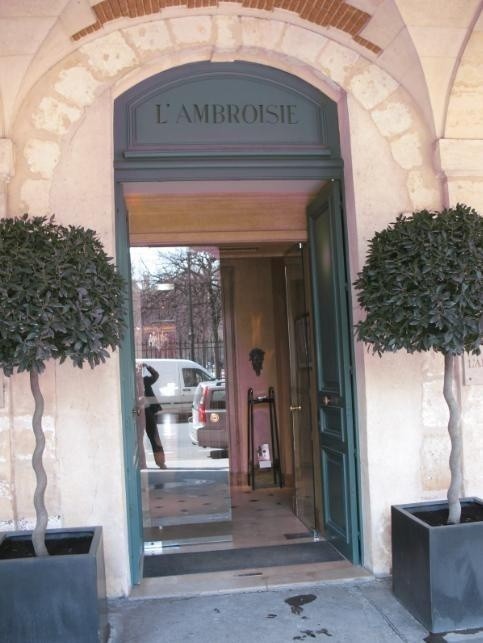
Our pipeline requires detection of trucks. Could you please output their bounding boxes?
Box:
[135,358,218,415]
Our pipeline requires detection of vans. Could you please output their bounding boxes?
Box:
[190,378,229,450]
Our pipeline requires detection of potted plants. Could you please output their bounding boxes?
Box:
[0,211,131,642]
[352,201,483,633]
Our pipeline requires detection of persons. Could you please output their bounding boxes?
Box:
[141,363,168,471]
